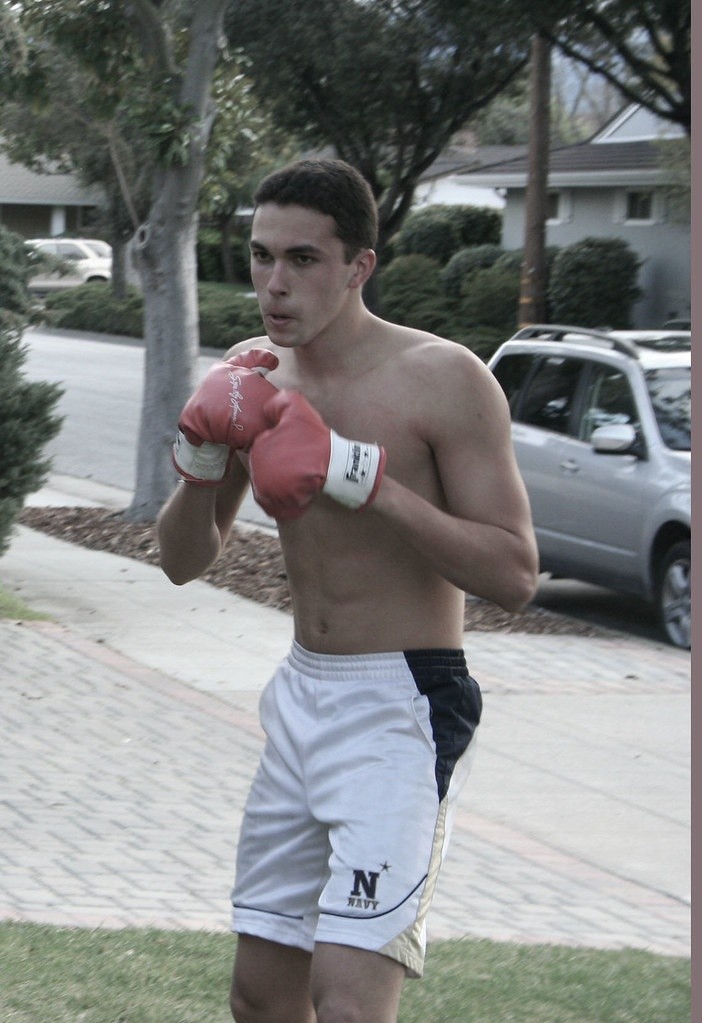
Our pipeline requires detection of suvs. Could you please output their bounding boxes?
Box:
[481,324,693,652]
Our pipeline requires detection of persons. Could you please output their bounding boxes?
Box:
[158,159,540,1022]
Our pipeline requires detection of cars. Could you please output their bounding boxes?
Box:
[19,238,113,296]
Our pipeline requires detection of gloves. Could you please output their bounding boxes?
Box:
[248,388,387,520]
[172,348,280,486]
